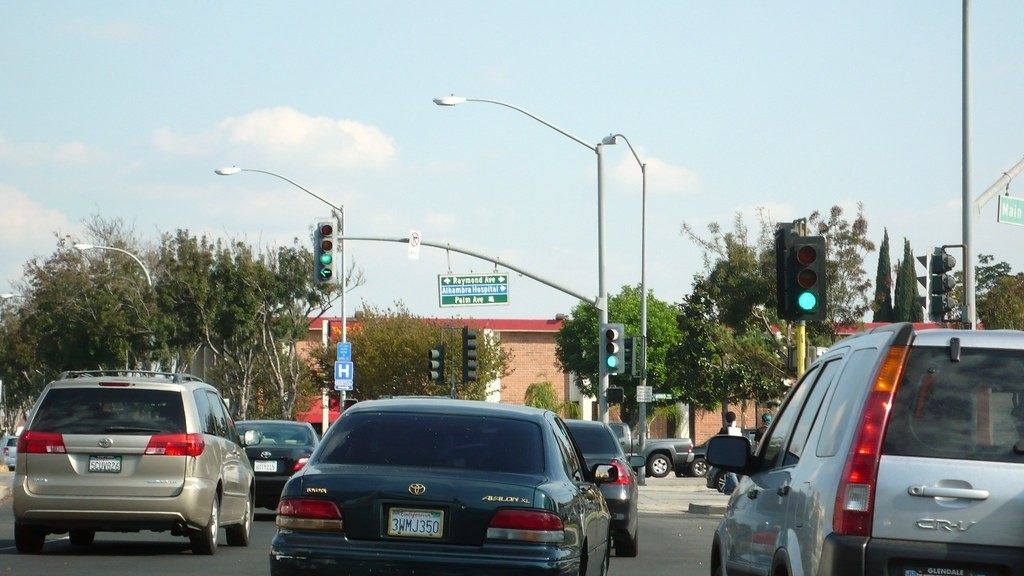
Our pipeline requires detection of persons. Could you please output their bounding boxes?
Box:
[719,411,741,495]
[754,413,772,451]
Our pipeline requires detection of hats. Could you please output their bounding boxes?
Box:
[761,413,772,425]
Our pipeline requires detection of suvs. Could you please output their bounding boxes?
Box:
[13,369,257,553]
[704,322,1023,576]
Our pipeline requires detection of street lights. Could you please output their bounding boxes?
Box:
[215,165,350,414]
[432,93,609,428]
[73,243,156,375]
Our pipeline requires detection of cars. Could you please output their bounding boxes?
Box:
[691,428,763,478]
[268,396,619,576]
[232,419,319,512]
[562,419,647,557]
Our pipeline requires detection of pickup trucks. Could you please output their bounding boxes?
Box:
[608,422,695,478]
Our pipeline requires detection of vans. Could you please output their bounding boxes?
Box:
[3,436,20,472]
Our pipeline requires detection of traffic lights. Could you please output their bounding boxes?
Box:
[914,253,956,323]
[316,221,339,286]
[790,235,827,323]
[462,326,478,383]
[600,322,625,374]
[428,344,444,384]
